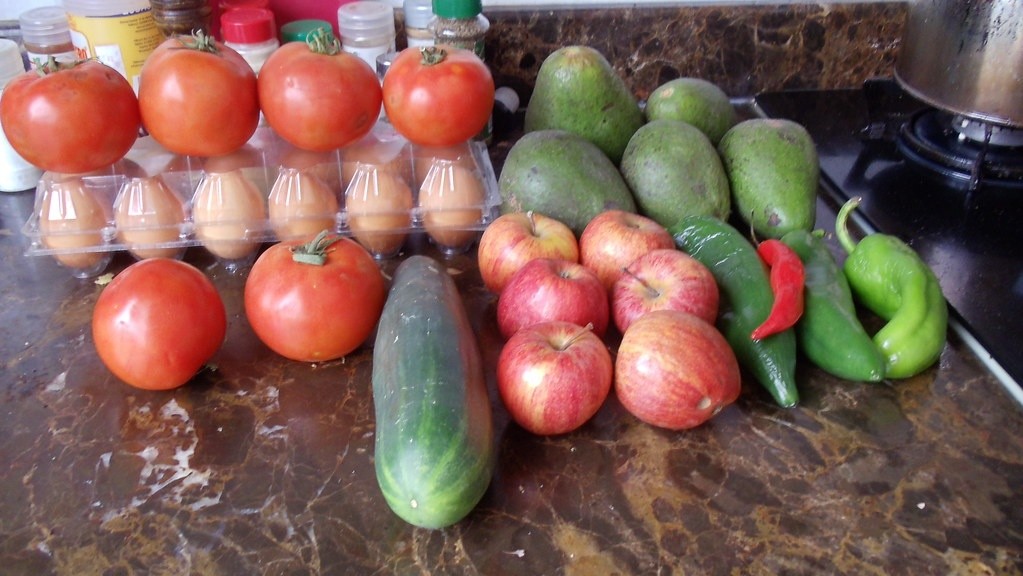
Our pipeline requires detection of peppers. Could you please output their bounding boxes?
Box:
[671,195,948,409]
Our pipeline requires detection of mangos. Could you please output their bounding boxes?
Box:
[492,43,818,239]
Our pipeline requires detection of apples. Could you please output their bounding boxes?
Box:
[479,209,742,436]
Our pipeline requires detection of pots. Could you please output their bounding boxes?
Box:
[891,0,1023,132]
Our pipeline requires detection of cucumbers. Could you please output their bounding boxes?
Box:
[372,254,494,529]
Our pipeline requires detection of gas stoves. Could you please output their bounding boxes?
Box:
[750,77,1023,408]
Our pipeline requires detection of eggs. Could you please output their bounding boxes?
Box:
[38,129,489,268]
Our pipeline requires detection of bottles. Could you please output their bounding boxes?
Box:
[0,0,492,193]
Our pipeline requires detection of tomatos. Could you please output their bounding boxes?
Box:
[137,26,261,157]
[91,256,227,390]
[0,52,140,173]
[380,43,494,149]
[256,28,382,153]
[243,229,387,364]
